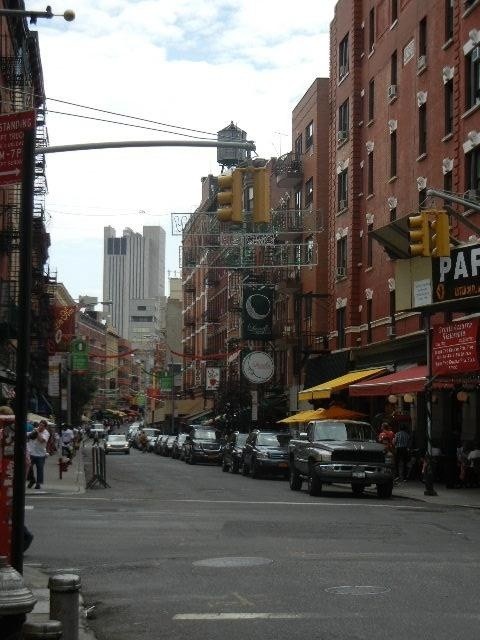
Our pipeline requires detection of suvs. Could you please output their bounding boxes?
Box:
[89,423,106,437]
[242,429,291,479]
[184,427,222,465]
[222,430,249,472]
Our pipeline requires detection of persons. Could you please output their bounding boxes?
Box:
[392,422,413,484]
[376,421,399,486]
[466,442,480,459]
[25,419,86,490]
[426,440,443,456]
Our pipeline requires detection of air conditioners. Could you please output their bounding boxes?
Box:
[388,85,396,97]
[416,56,425,69]
[337,131,348,142]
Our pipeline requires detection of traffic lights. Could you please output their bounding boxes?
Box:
[408,215,422,254]
[216,174,233,221]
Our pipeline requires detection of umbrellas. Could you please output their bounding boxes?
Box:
[275,405,370,435]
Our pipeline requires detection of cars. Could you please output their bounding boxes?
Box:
[127,421,188,459]
[103,434,129,455]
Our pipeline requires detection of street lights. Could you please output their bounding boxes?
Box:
[424,211,441,258]
[136,355,176,435]
[53,299,114,338]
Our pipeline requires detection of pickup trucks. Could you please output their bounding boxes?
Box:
[289,420,394,498]
